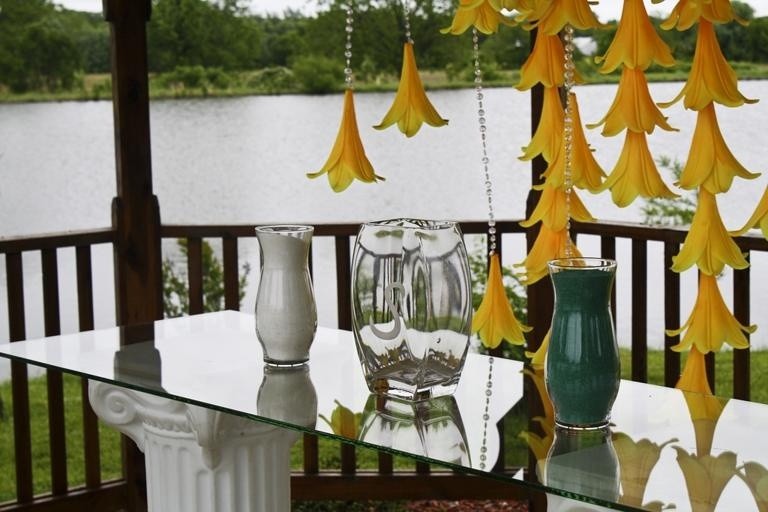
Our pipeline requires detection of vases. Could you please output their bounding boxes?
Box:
[352,218,473,395]
[251,224,319,368]
[545,258,623,434]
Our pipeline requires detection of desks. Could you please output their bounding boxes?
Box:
[1,309,768,511]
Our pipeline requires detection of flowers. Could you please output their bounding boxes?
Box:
[315,394,376,442]
[305,0,768,422]
[515,367,768,511]
